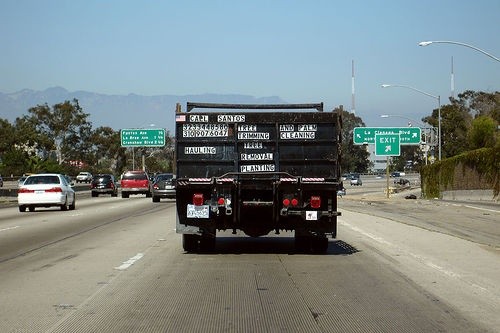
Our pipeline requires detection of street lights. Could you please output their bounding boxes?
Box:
[379,84,441,162]
[380,115,427,146]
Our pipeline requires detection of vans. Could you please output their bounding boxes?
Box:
[390,172,400,178]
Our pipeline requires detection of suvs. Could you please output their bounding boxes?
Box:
[76,171,93,184]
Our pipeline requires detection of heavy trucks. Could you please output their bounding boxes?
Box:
[173,99,347,254]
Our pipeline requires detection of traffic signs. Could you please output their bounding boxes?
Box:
[374,133,402,157]
[352,127,422,147]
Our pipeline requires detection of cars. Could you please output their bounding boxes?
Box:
[0,173,3,187]
[341,174,363,186]
[90,173,118,197]
[151,173,176,202]
[16,173,76,212]
[120,170,152,198]
